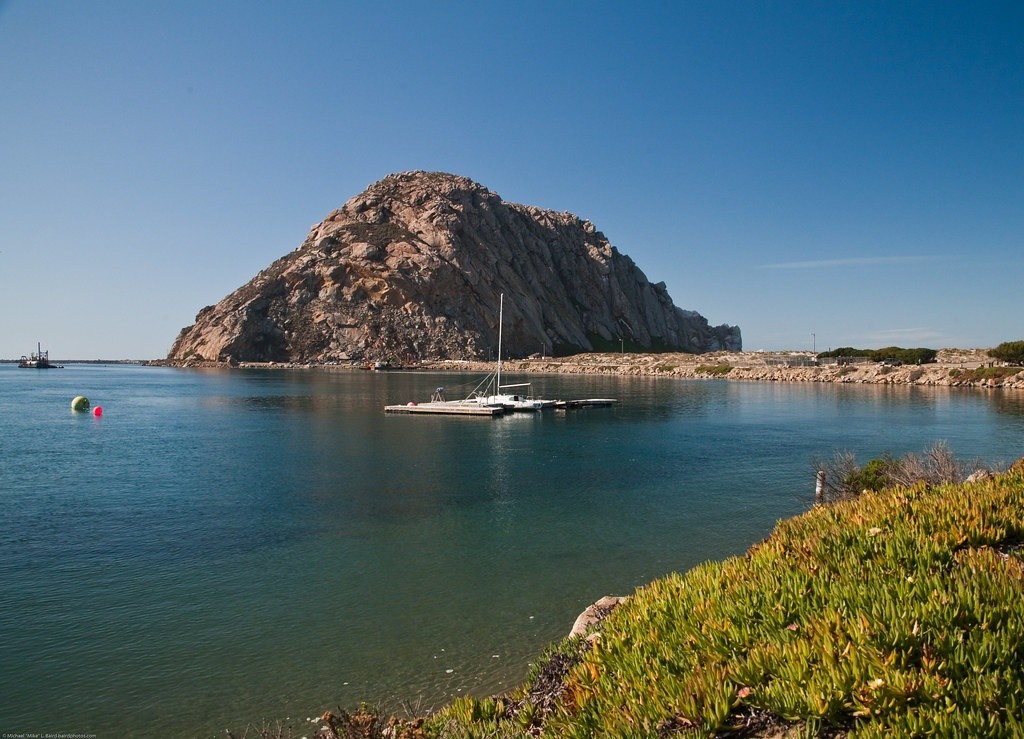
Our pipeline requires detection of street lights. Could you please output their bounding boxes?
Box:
[811,333,816,367]
[619,339,624,357]
[541,342,546,357]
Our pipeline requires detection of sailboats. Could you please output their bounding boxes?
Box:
[417,293,543,410]
[17,341,58,369]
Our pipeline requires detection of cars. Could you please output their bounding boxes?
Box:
[879,358,897,366]
